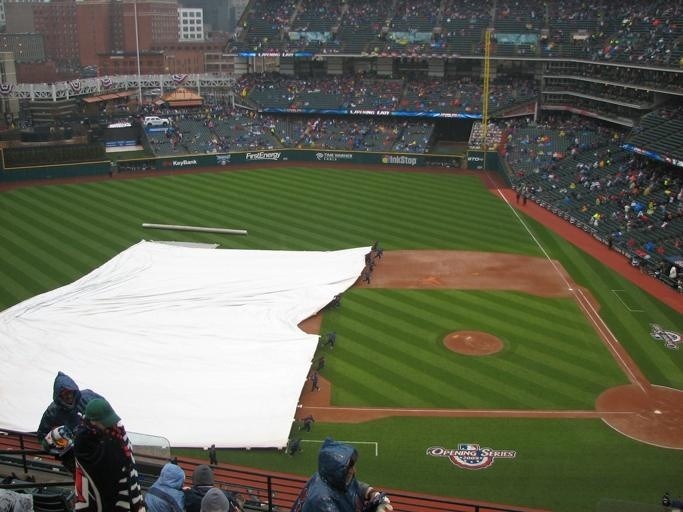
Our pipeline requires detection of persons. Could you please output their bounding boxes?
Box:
[206,444,218,466]
[144,460,186,512]
[183,463,245,512]
[309,372,321,392]
[288,436,304,456]
[298,414,315,432]
[51,1,682,294]
[330,296,340,309]
[319,331,336,350]
[246,494,261,507]
[37,370,149,512]
[277,448,283,456]
[314,356,324,371]
[663,493,682,512]
[290,437,394,512]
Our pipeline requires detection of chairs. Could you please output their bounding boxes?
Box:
[29,489,71,511]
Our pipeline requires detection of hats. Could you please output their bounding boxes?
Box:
[84,398,121,428]
